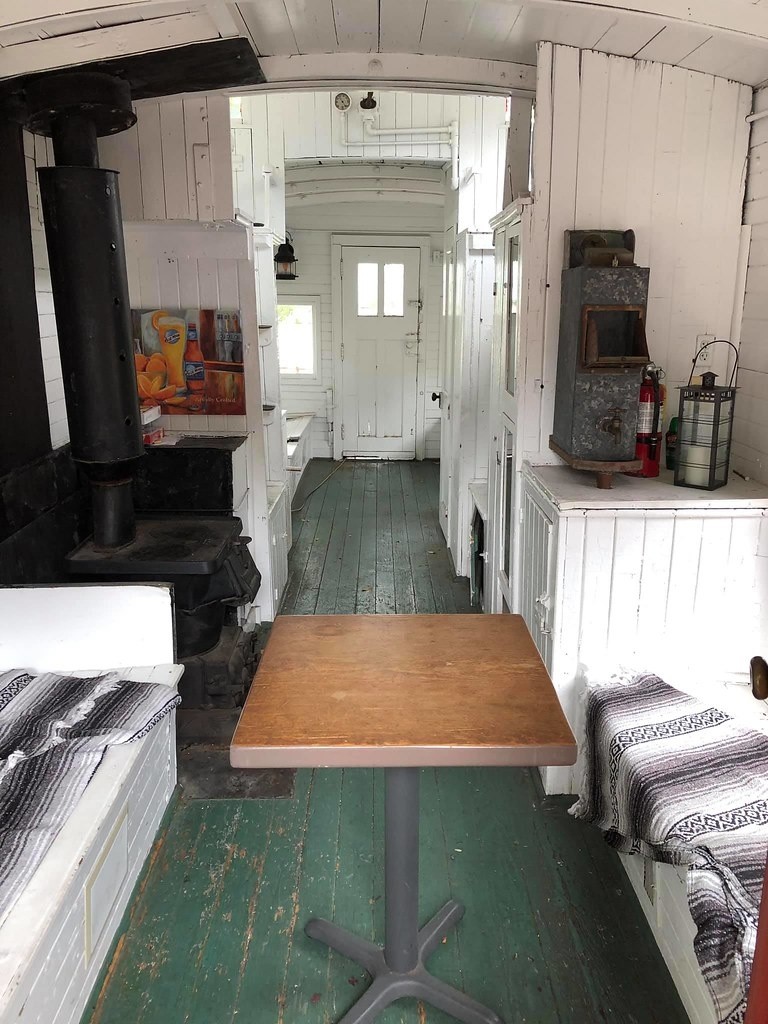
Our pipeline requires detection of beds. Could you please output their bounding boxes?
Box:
[0,585,182,1024]
[580,668,768,1024]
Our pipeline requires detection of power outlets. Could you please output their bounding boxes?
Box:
[696,336,716,367]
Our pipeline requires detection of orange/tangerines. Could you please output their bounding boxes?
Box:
[135,352,186,405]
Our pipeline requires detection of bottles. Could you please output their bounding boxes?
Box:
[183,321,208,412]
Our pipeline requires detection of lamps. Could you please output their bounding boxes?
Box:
[274,231,299,280]
[674,340,741,491]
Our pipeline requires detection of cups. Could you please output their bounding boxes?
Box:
[158,317,187,393]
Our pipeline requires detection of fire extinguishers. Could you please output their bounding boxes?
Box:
[622,364,666,478]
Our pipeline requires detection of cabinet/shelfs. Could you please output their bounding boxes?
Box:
[518,473,768,796]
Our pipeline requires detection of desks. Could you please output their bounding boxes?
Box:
[230,614,577,1024]
